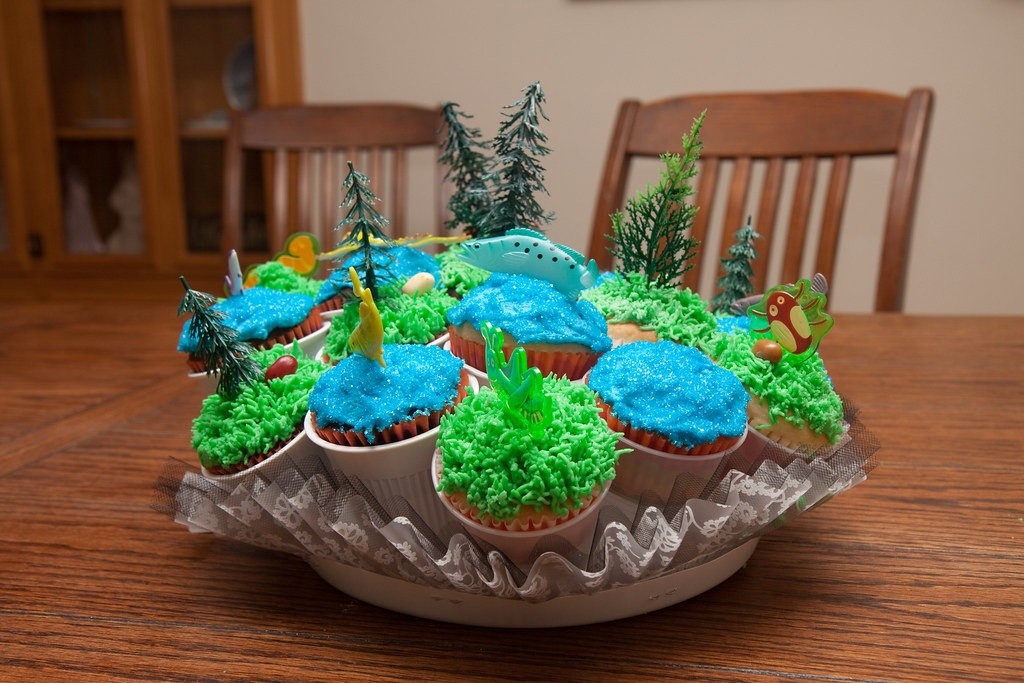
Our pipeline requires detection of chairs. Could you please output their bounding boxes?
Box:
[224,104,449,276]
[585,87,936,313]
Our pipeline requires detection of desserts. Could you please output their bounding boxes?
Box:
[177,231,845,570]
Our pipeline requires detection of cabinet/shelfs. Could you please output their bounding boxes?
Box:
[0,1,305,275]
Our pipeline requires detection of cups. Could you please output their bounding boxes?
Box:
[186,304,840,567]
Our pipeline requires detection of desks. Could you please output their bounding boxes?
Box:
[1,312,1024,683]
